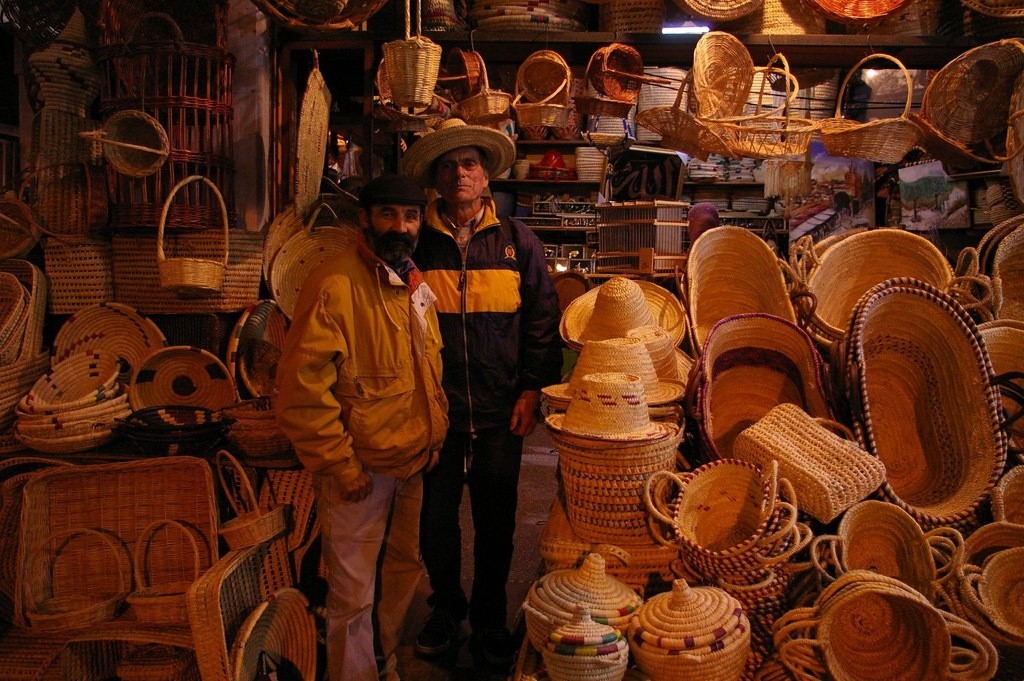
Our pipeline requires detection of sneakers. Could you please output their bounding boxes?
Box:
[414,606,465,653]
[468,608,515,664]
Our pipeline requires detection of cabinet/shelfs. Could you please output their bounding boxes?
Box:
[488,138,788,278]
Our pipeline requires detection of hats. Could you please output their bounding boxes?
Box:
[360,174,430,207]
[542,276,696,450]
[400,119,515,184]
[527,150,573,179]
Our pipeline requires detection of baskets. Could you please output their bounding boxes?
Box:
[507,402,691,681]
[120,12,182,98]
[645,216,1024,681]
[0,69,368,681]
[377,0,1024,211]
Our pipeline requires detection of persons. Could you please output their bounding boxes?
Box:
[274,175,449,681]
[401,119,563,662]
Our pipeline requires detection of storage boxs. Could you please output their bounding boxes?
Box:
[542,243,593,275]
